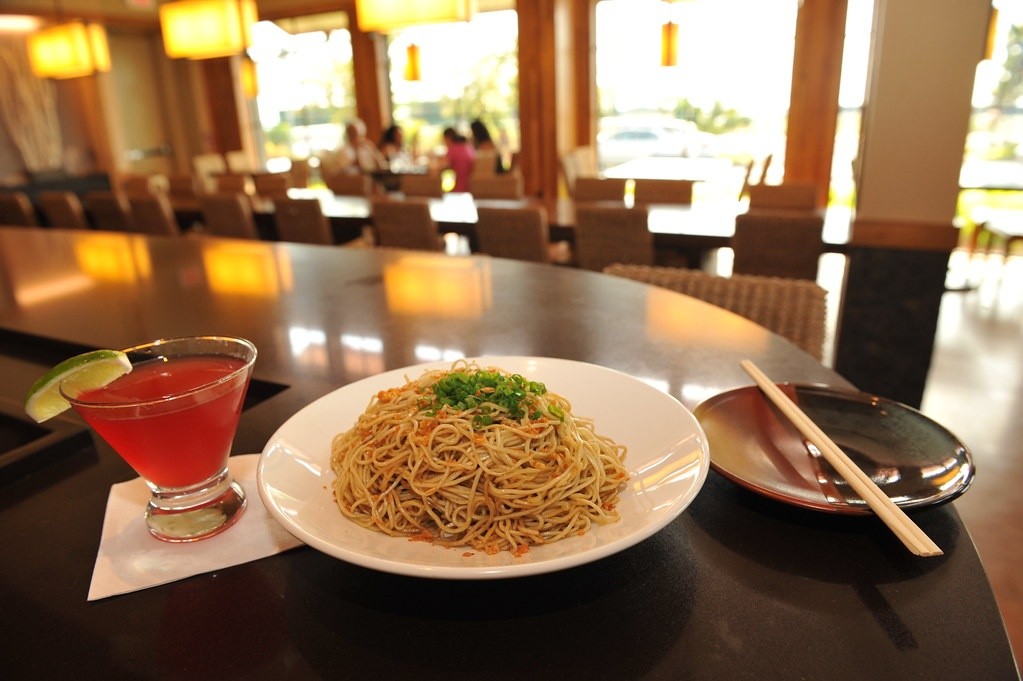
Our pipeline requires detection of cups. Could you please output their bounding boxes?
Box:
[60,333,259,542]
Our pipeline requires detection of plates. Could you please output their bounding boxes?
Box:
[258,355,709,579]
[692,383,977,513]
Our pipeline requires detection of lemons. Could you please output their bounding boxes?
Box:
[25,350,133,423]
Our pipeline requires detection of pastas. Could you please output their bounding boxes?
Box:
[328,358,631,555]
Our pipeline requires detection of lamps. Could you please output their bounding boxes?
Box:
[155,0,260,61]
[30,17,110,84]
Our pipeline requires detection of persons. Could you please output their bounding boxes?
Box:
[439,117,506,193]
[341,119,405,192]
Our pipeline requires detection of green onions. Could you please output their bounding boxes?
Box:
[419,369,566,430]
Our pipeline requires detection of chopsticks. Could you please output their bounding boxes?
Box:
[739,358,942,556]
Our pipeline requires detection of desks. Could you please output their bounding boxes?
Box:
[124,187,955,270]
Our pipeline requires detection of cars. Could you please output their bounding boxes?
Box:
[597,111,714,164]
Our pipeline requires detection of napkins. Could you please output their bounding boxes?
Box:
[88,455,315,602]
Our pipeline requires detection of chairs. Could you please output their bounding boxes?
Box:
[574,200,650,270]
[578,178,697,203]
[732,205,827,283]
[749,183,826,210]
[471,178,518,198]
[276,195,338,245]
[4,173,297,242]
[469,148,499,186]
[373,195,441,253]
[476,199,550,261]
[405,172,445,198]
[342,177,373,194]
